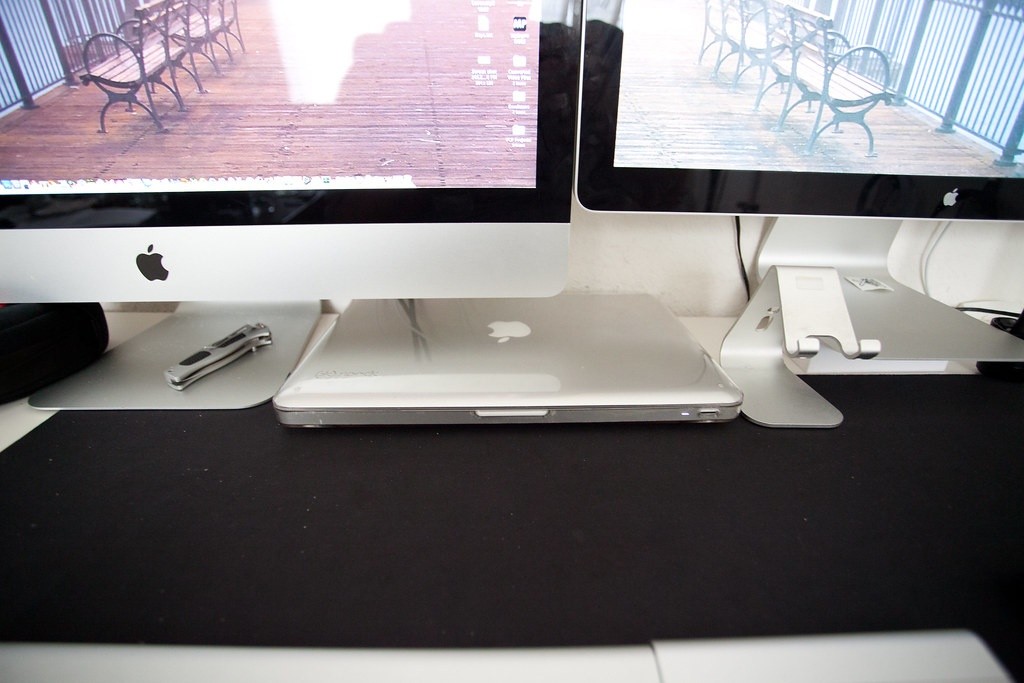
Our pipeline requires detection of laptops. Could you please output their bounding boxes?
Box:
[272,298,744,426]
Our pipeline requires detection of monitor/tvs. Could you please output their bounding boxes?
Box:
[0,0,1024,409]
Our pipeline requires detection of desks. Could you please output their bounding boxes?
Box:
[0,315,1024,683]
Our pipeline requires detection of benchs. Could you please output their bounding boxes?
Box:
[78,1,247,134]
[697,0,896,157]
